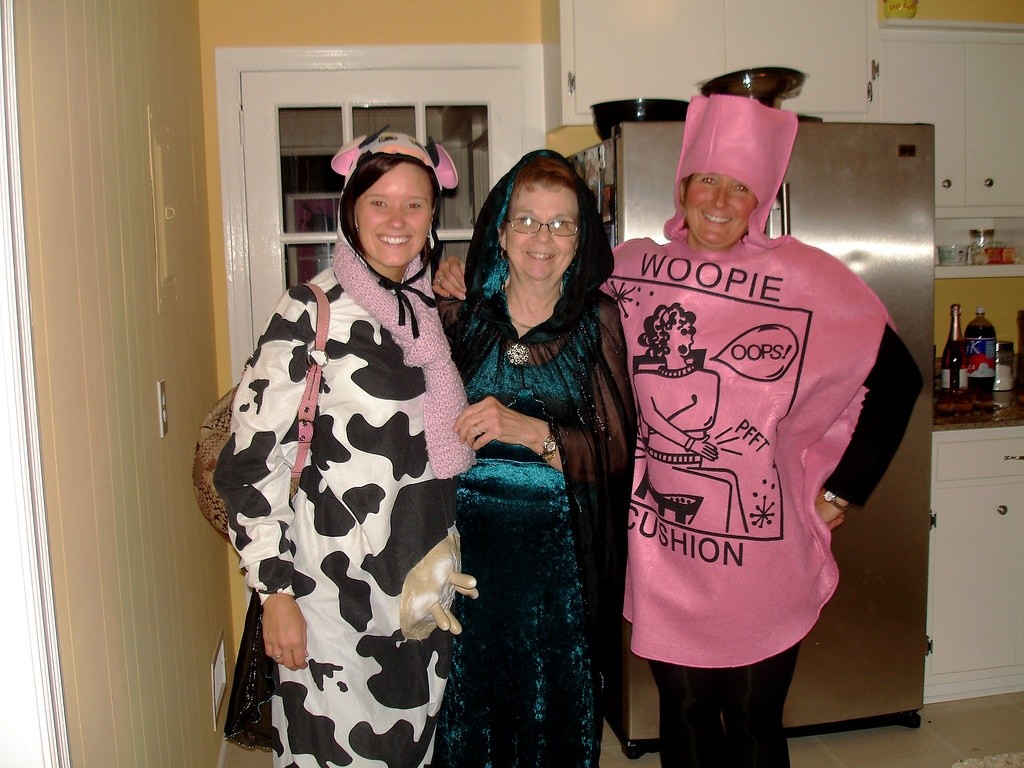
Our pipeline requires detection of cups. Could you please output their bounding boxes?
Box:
[941,304,970,396]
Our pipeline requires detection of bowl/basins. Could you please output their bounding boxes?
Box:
[589,99,692,142]
[936,244,972,266]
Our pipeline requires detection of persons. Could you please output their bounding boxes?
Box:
[431,95,924,768]
[225,132,474,768]
[429,150,636,768]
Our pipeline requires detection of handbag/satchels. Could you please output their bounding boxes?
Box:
[192,282,330,543]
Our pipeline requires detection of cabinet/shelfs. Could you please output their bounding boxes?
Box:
[923,427,1024,705]
[561,0,1024,218]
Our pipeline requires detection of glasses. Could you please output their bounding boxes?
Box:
[504,217,580,237]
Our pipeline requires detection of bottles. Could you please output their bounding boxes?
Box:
[969,229,994,266]
[964,307,996,397]
[994,340,1015,391]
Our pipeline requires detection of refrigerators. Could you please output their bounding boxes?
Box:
[565,112,935,761]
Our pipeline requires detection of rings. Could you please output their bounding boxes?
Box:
[437,277,444,285]
[273,654,282,660]
[474,424,482,434]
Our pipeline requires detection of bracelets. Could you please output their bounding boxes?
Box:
[545,431,562,463]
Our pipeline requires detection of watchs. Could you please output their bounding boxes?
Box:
[537,422,556,457]
[823,491,850,511]
